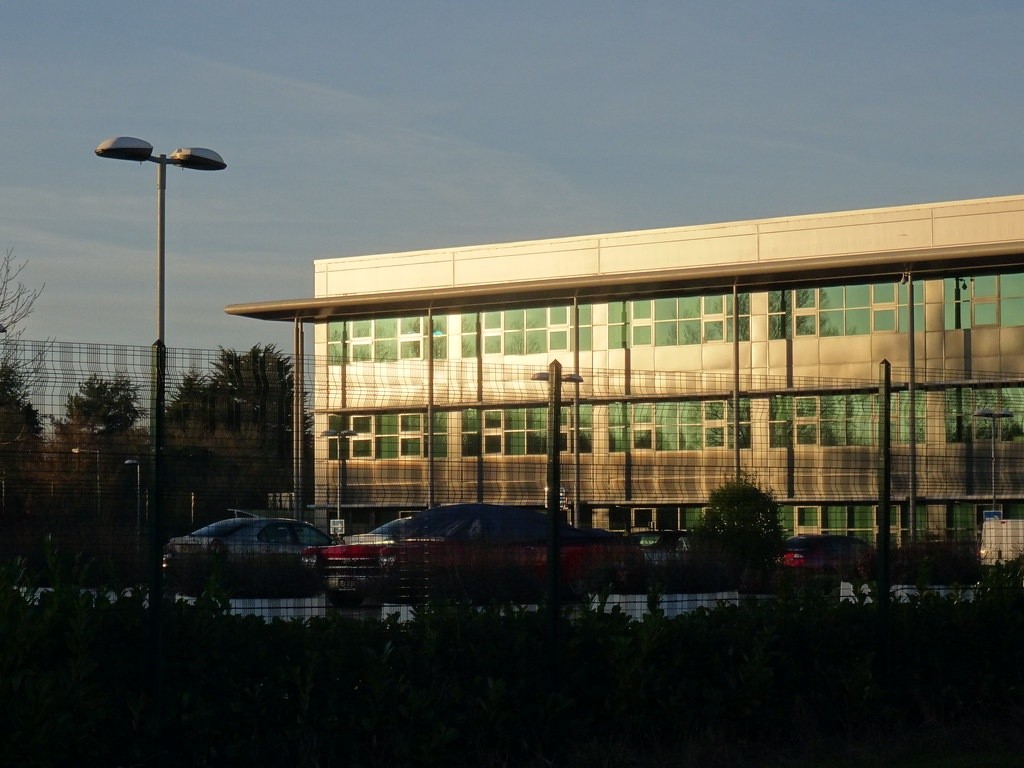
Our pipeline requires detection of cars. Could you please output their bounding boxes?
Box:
[765,533,878,596]
[163,516,342,598]
[302,503,646,607]
[614,528,725,595]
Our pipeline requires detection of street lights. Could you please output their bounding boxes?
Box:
[530,359,585,645]
[973,406,1012,510]
[92,136,229,662]
